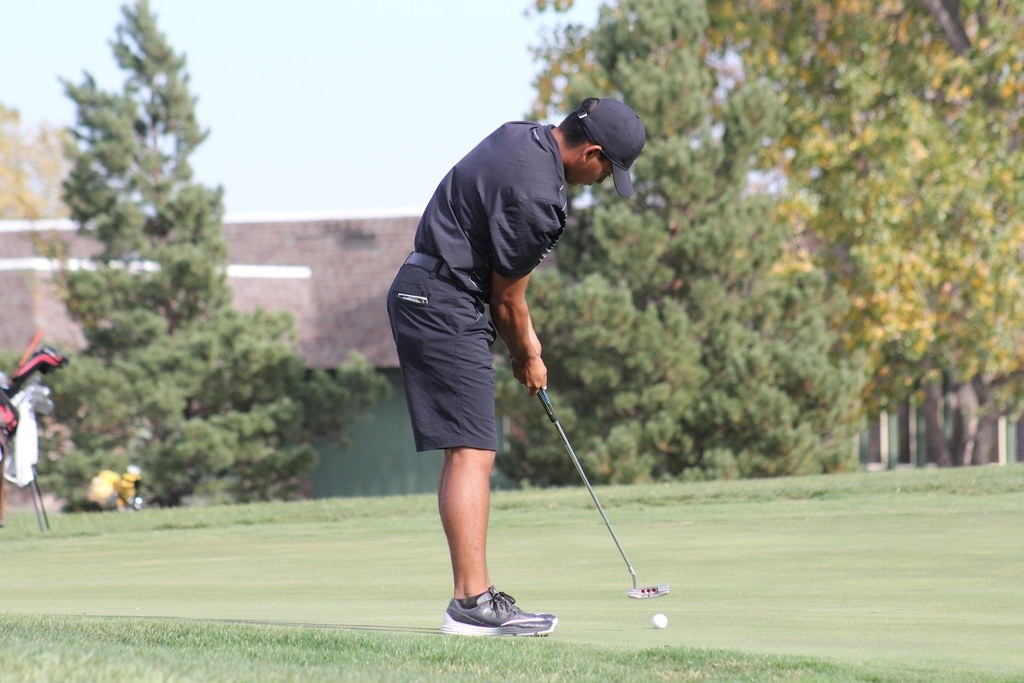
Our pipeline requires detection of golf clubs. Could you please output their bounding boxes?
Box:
[9,384,55,416]
[537,387,671,600]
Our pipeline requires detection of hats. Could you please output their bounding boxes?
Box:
[576,99,646,197]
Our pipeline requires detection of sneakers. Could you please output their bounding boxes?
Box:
[440,584,556,636]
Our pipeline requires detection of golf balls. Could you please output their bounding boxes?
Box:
[652,614,668,629]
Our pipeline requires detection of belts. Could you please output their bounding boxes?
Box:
[406,251,460,283]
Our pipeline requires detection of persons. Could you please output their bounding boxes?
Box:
[386,97,646,636]
